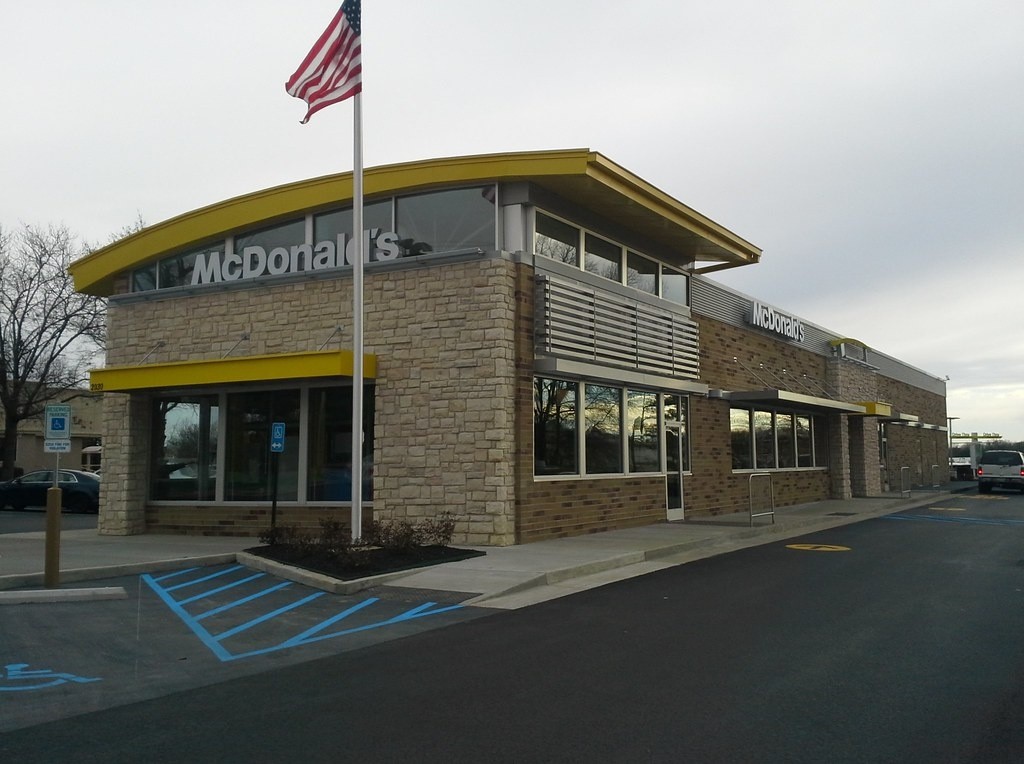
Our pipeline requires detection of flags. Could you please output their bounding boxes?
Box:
[284,0,363,124]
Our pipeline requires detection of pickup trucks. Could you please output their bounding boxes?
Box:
[976,449,1024,494]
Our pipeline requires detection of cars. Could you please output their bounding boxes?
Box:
[0,468,100,514]
